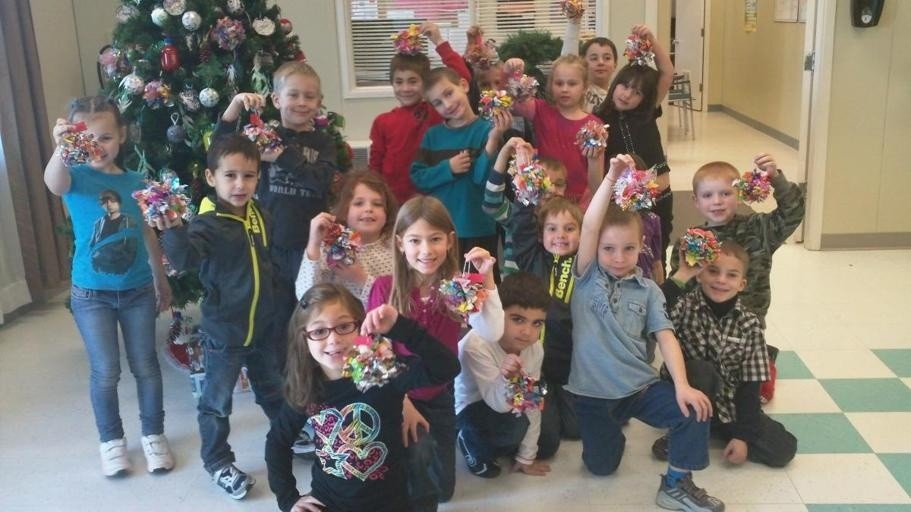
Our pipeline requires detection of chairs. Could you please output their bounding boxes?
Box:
[667,66,697,144]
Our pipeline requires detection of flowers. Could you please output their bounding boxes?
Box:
[560,0,585,23]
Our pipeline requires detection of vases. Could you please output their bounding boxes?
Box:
[560,17,583,58]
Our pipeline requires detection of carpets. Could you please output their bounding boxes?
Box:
[761,346,910,511]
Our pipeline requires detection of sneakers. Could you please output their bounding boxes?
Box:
[456,428,503,480]
[208,461,259,501]
[291,429,317,455]
[140,432,175,475]
[654,472,726,512]
[99,436,134,478]
[651,434,669,461]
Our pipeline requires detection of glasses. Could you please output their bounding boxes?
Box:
[303,319,359,341]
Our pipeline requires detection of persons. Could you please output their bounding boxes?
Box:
[89,190,138,276]
[294,166,401,310]
[666,150,806,406]
[561,152,726,512]
[206,60,353,297]
[264,283,463,512]
[152,130,316,500]
[452,269,561,478]
[364,20,674,438]
[41,94,176,481]
[650,227,799,467]
[362,195,506,512]
